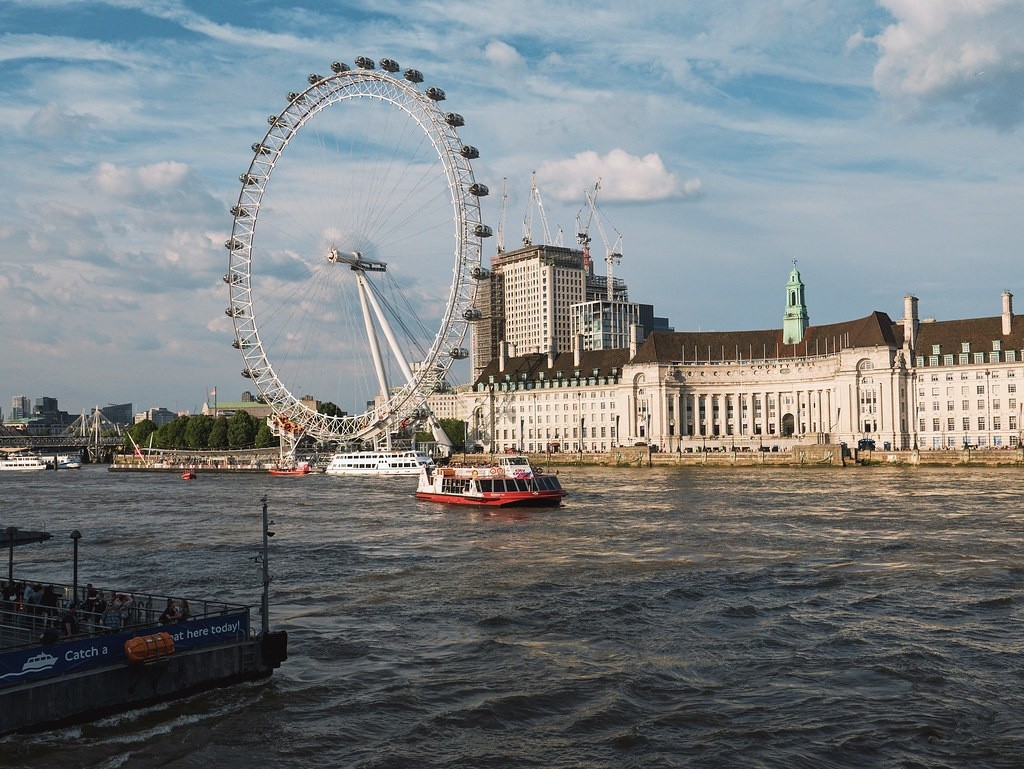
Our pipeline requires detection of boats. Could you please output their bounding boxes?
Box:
[267,468,308,476]
[324,450,435,475]
[38,454,82,470]
[177,469,197,480]
[415,448,569,508]
[0,455,47,471]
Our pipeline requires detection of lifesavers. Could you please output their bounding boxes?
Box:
[490,468,496,474]
[472,470,478,475]
[537,468,543,473]
[519,468,525,474]
[497,468,503,473]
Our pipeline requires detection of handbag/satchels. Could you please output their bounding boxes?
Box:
[9,592,17,601]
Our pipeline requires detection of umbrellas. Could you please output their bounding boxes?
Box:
[1,526,52,580]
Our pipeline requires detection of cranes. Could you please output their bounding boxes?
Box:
[583,188,623,301]
[521,171,536,248]
[575,178,601,276]
[532,183,553,246]
[495,177,508,253]
[553,223,564,246]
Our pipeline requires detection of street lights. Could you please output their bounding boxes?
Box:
[616,415,620,442]
[6,526,19,581]
[581,417,585,449]
[247,494,275,633]
[69,529,83,605]
[520,419,524,452]
[532,392,539,454]
[576,391,584,453]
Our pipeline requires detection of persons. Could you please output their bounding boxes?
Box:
[2,580,190,645]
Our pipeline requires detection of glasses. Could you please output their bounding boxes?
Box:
[108,605,113,607]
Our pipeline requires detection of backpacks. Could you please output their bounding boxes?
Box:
[118,602,129,618]
